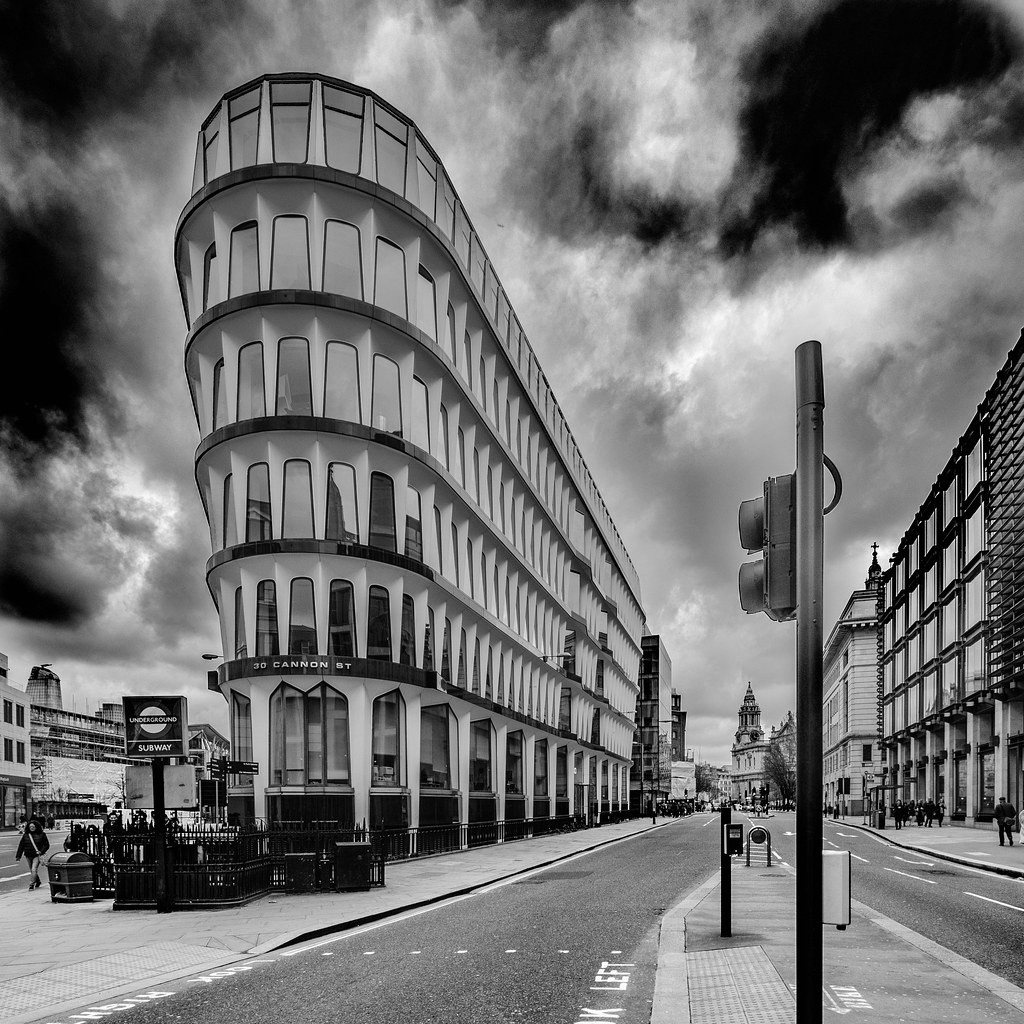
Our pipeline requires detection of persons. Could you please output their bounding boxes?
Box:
[995,797,1016,846]
[893,798,947,830]
[19,812,54,834]
[103,810,172,872]
[661,798,704,818]
[15,821,50,891]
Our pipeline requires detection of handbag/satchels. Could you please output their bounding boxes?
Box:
[38,853,50,866]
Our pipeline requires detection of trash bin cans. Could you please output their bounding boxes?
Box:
[876,812,885,829]
[334,841,372,892]
[285,852,316,893]
[318,860,332,893]
[45,850,95,903]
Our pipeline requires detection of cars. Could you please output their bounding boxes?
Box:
[712,807,721,812]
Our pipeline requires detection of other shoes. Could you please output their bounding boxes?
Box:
[29,885,34,891]
[35,882,41,888]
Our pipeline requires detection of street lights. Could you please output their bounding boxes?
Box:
[633,742,644,819]
[841,769,845,820]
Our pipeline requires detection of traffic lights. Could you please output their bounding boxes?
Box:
[684,790,688,796]
[737,473,799,623]
